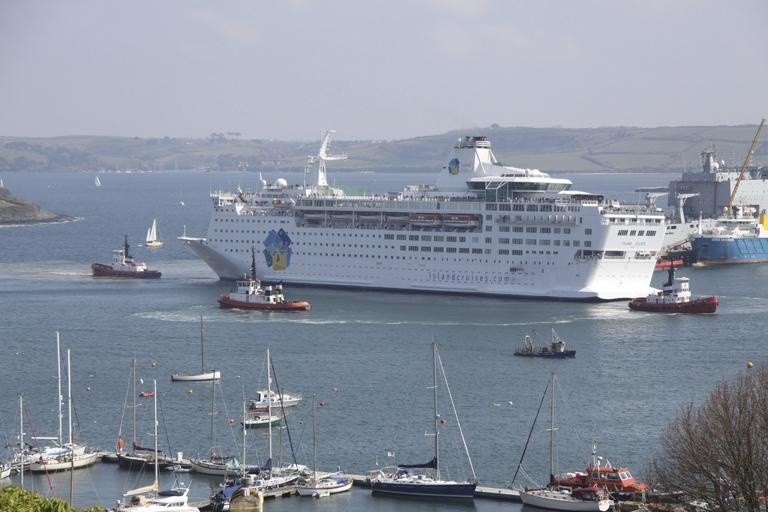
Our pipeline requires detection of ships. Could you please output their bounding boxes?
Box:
[652,117,768,266]
[176,126,669,307]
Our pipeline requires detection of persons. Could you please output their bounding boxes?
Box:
[117,435,125,455]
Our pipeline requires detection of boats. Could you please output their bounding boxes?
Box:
[636,184,670,192]
[627,262,720,315]
[85,234,166,281]
[218,244,313,315]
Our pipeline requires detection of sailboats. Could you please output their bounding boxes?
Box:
[94,175,102,188]
[143,218,164,249]
